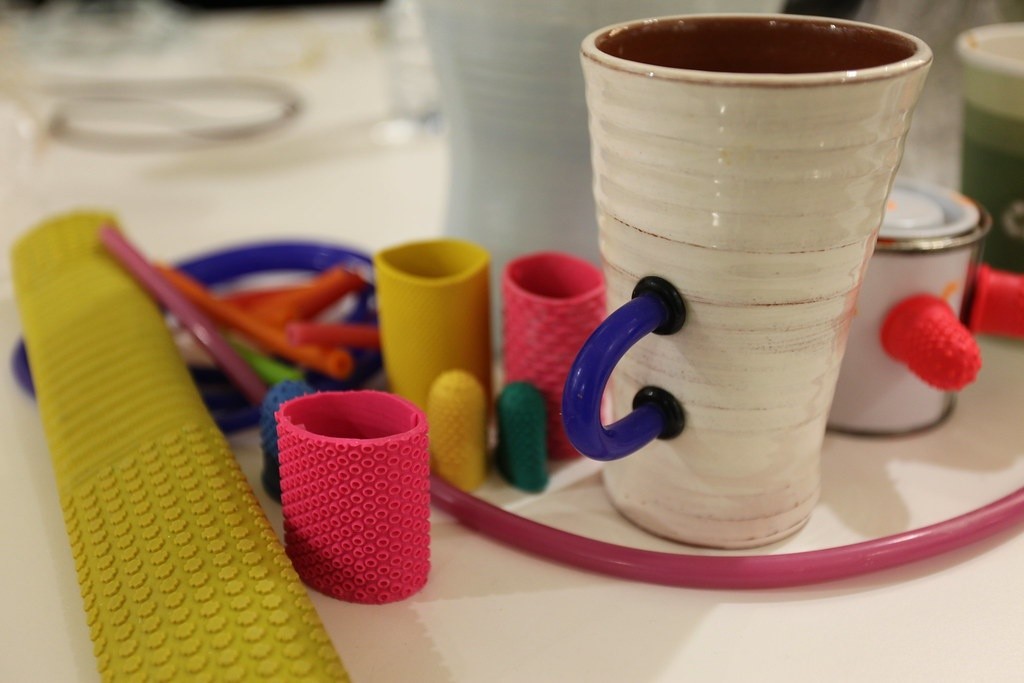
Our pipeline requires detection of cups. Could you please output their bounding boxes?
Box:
[419,0,788,361]
[826,179,993,434]
[956,22,1024,273]
[563,14,935,548]
